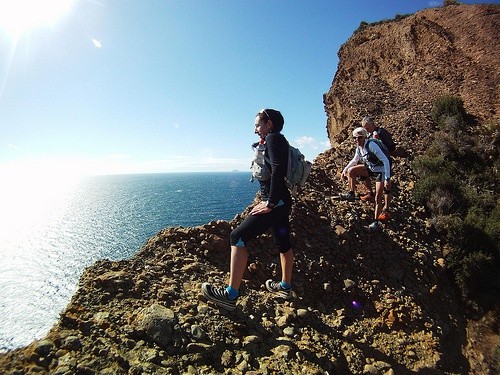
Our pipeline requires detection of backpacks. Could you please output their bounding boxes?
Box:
[284,143,313,202]
[376,127,396,153]
[365,139,392,166]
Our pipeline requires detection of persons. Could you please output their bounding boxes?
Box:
[201,110,292,311]
[339,117,392,229]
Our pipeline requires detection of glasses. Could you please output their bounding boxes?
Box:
[353,136,363,139]
[259,109,270,120]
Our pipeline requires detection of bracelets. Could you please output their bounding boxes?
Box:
[385,178,390,181]
[265,199,274,209]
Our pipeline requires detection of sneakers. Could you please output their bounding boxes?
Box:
[339,193,355,202]
[369,221,379,229]
[201,281,239,312]
[360,194,375,203]
[377,212,392,220]
[265,279,292,299]
